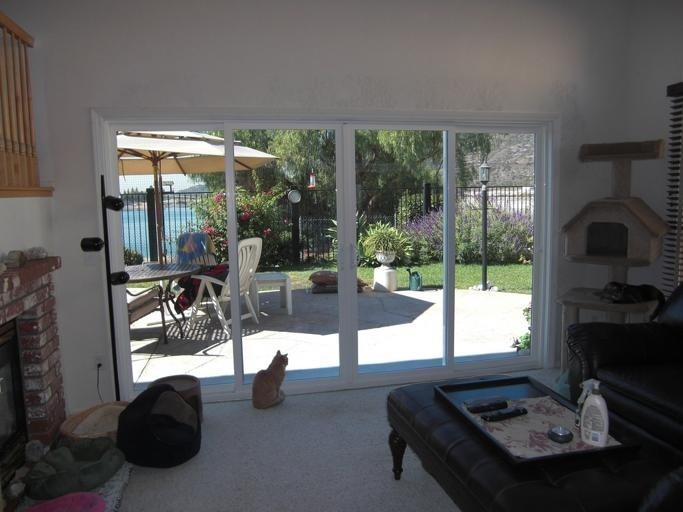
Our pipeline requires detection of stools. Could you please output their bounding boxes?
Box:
[152,375,203,427]
[250,272,293,317]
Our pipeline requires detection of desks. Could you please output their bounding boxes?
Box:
[125,264,202,339]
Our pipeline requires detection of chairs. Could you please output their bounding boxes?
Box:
[127,285,169,345]
[191,237,262,337]
[180,232,218,266]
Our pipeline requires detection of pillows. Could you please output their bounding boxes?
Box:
[309,271,369,287]
[311,283,362,293]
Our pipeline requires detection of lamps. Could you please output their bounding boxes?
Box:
[81,172,130,406]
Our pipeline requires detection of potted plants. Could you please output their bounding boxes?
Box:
[359,220,418,268]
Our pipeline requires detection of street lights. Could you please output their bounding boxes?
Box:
[477,155,491,291]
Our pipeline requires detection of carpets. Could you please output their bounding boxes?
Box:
[15,461,134,512]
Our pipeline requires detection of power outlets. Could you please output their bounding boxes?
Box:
[95,355,105,371]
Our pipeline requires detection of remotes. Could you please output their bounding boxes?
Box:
[481,406,527,421]
[467,399,507,413]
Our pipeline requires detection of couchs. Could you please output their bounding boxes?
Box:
[567,284,683,462]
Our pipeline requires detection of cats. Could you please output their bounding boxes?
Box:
[251,350,289,410]
[600,282,666,321]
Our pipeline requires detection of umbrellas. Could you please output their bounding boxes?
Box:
[115,132,282,285]
